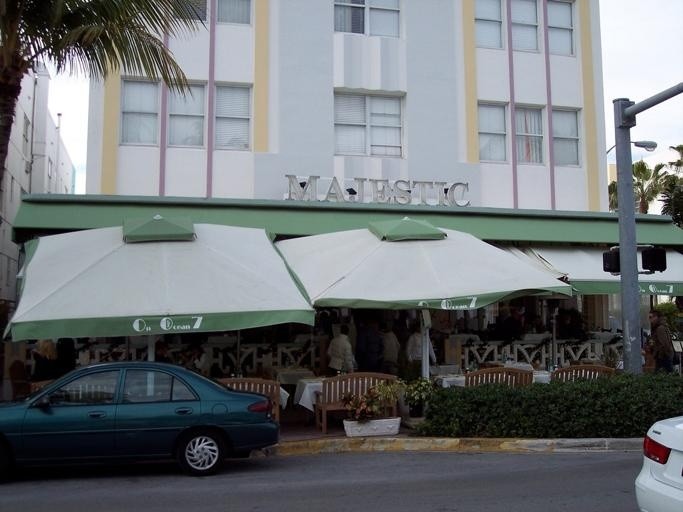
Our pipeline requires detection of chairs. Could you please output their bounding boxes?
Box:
[10,360,30,400]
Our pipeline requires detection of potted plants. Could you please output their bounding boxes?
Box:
[404,377,433,417]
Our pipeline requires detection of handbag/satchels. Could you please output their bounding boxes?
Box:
[672,355,679,365]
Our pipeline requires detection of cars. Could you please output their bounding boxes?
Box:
[0,360,280,478]
[632,415,682,511]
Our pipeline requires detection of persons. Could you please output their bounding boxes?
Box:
[30,339,78,382]
[80,335,258,383]
[468,299,586,348]
[644,311,680,374]
[317,308,436,382]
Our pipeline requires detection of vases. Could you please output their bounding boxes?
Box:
[343,416,402,437]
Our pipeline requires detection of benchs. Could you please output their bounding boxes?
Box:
[31,379,117,402]
[552,365,615,383]
[315,372,397,432]
[218,378,280,424]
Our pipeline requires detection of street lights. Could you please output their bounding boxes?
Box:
[605,139,656,156]
